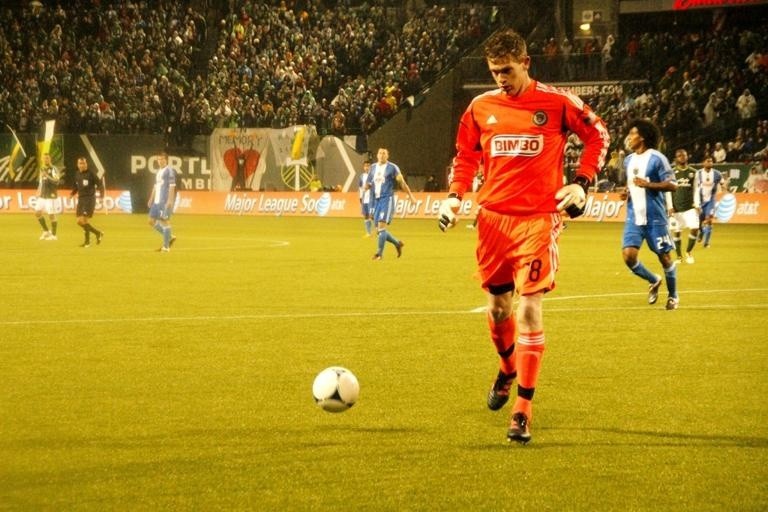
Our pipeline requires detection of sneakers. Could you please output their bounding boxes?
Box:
[649,276,678,309]
[80,232,103,247]
[372,241,402,259]
[40,231,57,240]
[488,369,531,442]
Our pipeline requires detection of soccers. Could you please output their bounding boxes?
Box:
[312,367,358,412]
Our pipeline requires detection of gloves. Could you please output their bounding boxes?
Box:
[439,192,461,232]
[554,176,590,218]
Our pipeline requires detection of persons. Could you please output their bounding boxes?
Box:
[621,120,678,310]
[438,32,611,444]
